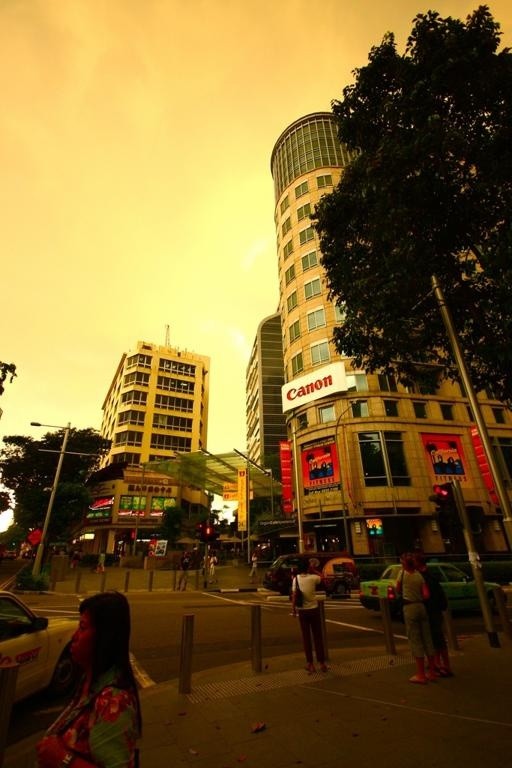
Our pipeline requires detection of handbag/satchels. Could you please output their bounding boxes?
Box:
[291,576,304,607]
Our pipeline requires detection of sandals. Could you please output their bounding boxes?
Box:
[304,664,332,672]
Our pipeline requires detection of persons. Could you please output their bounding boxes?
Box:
[248,550,261,585]
[394,552,439,686]
[93,548,105,574]
[201,552,210,574]
[71,547,80,568]
[413,550,454,678]
[175,550,192,592]
[33,590,142,767]
[290,558,329,673]
[208,551,218,585]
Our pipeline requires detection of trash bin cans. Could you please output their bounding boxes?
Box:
[51,555,69,581]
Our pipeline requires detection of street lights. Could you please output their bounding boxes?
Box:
[31,421,71,582]
[293,421,309,551]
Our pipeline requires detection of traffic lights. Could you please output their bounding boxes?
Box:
[430,481,464,538]
[202,525,214,542]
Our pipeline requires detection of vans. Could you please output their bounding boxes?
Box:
[263,551,351,594]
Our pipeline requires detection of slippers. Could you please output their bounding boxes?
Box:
[408,668,454,684]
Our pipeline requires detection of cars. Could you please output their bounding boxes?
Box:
[0,589,84,709]
[358,562,506,625]
